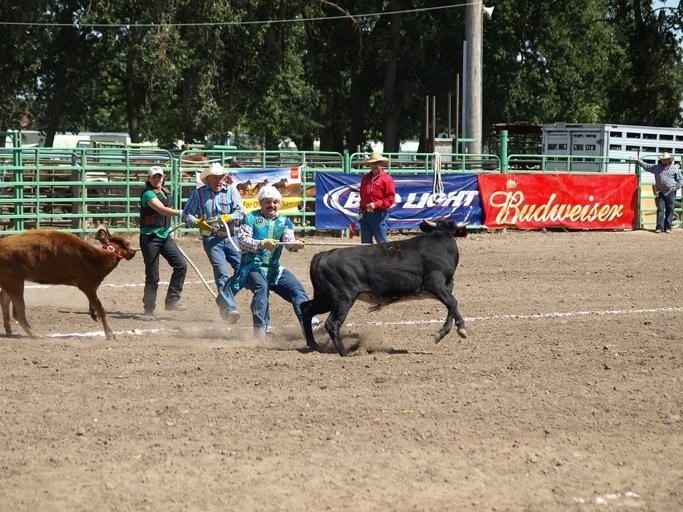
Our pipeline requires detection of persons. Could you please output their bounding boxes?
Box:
[632,151,683,233]
[137,165,190,318]
[358,150,396,244]
[180,160,248,324]
[236,183,324,342]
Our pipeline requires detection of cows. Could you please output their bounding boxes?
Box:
[42,179,121,226]
[0,225,142,341]
[301,218,472,358]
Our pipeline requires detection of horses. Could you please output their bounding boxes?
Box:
[132,152,209,198]
[253,179,269,193]
[236,180,252,195]
[272,178,288,193]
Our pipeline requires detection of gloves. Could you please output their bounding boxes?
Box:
[196,214,214,233]
[261,239,279,249]
[219,214,232,224]
[294,240,305,250]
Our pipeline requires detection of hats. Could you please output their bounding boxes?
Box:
[148,166,162,177]
[257,185,284,202]
[201,162,228,184]
[366,152,388,165]
[658,152,674,160]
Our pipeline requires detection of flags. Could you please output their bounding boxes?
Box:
[349,223,355,238]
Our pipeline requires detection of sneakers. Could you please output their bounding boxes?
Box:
[216,295,240,324]
[311,323,327,335]
[165,304,186,310]
